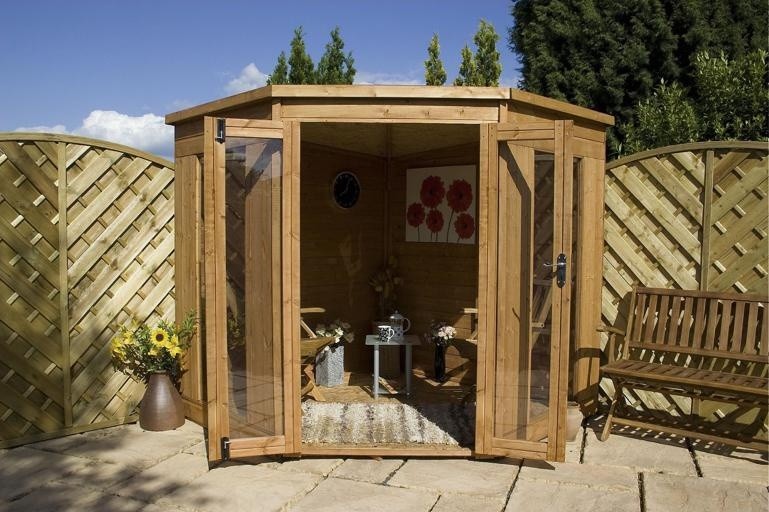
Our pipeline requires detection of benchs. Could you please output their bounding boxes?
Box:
[586,280,767,456]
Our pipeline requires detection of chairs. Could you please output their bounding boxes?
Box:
[295,305,335,406]
[454,265,576,403]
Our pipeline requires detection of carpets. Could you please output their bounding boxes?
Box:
[296,396,482,448]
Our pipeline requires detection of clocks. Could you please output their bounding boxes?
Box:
[330,168,363,211]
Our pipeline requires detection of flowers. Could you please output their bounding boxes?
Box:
[98,299,202,390]
[405,172,476,246]
[421,315,457,352]
[222,303,248,349]
[306,314,358,351]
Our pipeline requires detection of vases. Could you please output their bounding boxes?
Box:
[432,345,449,384]
[227,345,248,372]
[133,366,187,433]
[313,341,345,390]
[372,346,403,380]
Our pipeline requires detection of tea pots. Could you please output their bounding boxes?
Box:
[390,310,411,337]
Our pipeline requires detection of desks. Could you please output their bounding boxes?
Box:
[361,331,422,402]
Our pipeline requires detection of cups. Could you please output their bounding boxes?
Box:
[378,325,395,342]
